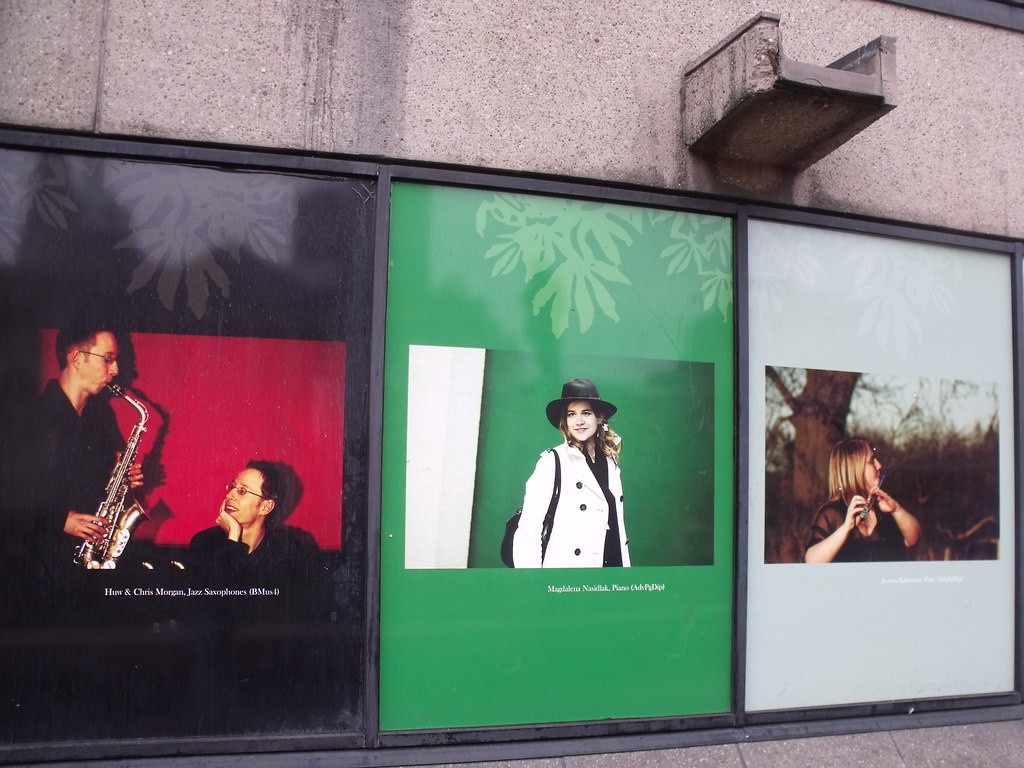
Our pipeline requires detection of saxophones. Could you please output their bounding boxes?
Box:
[73,381,153,569]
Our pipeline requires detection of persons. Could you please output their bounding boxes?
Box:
[801,437,921,563]
[513,377,634,568]
[0,316,198,620]
[181,461,351,733]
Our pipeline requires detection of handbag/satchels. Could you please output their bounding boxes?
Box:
[501,448,561,567]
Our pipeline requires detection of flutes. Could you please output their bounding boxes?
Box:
[858,467,886,523]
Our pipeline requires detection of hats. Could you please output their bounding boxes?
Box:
[545,378,618,428]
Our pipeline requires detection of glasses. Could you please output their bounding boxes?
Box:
[225,485,271,499]
[77,350,115,364]
